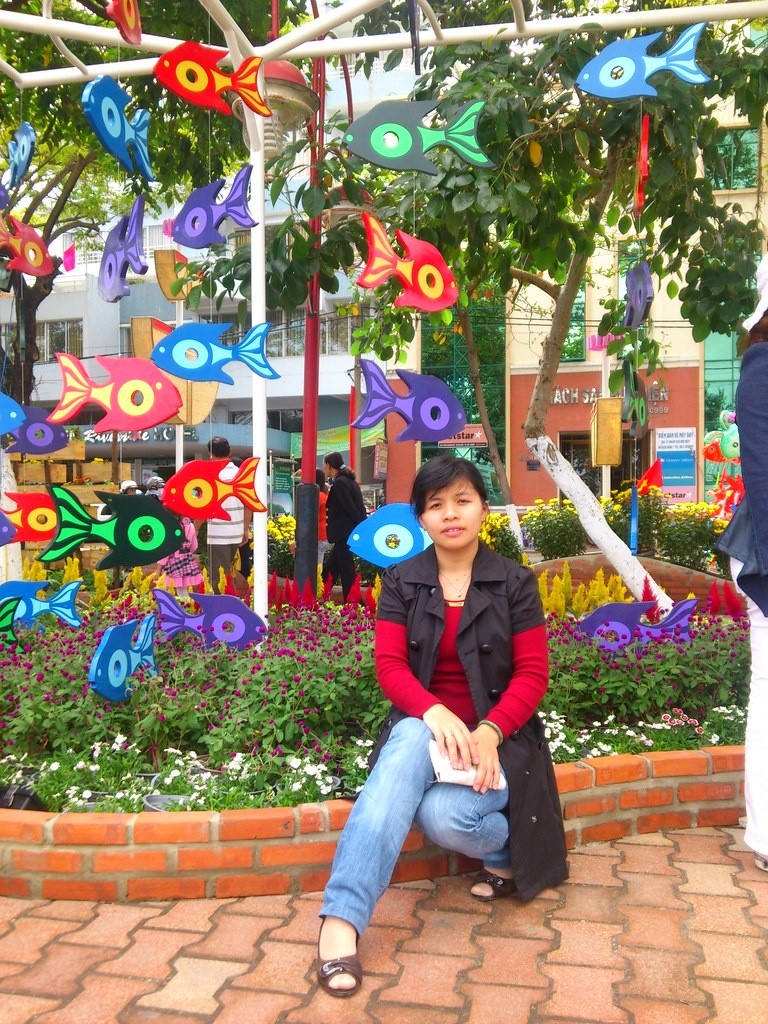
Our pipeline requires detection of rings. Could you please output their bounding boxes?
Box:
[445,735,454,738]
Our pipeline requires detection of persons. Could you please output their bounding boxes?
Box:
[715,253,767,875]
[315,456,570,999]
[117,437,368,606]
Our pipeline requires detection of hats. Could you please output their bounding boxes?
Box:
[741,251,768,332]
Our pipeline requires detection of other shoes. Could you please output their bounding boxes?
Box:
[754,851,768,872]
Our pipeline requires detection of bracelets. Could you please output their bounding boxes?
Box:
[477,720,503,745]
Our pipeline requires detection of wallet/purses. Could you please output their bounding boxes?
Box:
[429,739,506,790]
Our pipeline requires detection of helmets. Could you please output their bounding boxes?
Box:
[146,476,166,490]
[121,480,138,494]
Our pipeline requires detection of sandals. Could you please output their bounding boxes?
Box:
[470,867,517,902]
[318,915,362,997]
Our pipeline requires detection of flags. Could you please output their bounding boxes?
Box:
[636,459,661,495]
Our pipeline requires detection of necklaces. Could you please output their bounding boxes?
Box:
[440,567,471,598]
